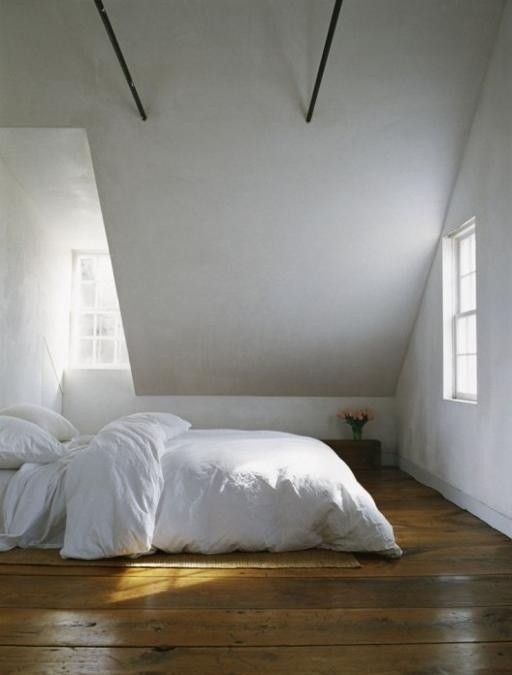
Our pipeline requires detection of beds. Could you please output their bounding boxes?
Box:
[1,400,405,561]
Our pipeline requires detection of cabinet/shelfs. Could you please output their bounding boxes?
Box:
[321,438,381,468]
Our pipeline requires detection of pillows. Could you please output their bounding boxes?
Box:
[0,414,70,473]
[1,402,79,444]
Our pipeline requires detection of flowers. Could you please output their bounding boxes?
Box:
[338,406,372,439]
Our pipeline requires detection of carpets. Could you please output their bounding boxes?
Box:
[1,544,362,569]
